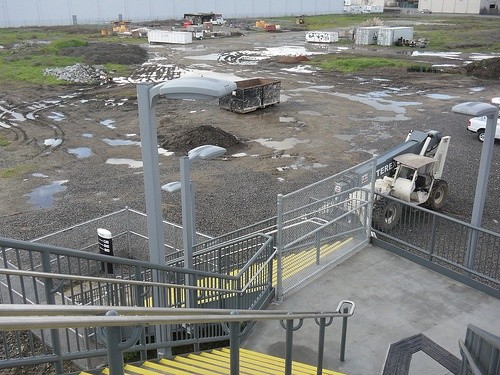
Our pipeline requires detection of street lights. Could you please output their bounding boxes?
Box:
[451,102,499,271]
[134,76,236,358]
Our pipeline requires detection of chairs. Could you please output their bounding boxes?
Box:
[413,175,427,192]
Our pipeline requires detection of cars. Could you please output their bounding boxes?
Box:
[467,98,500,144]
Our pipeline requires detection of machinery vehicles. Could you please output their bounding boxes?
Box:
[335,128,447,234]
